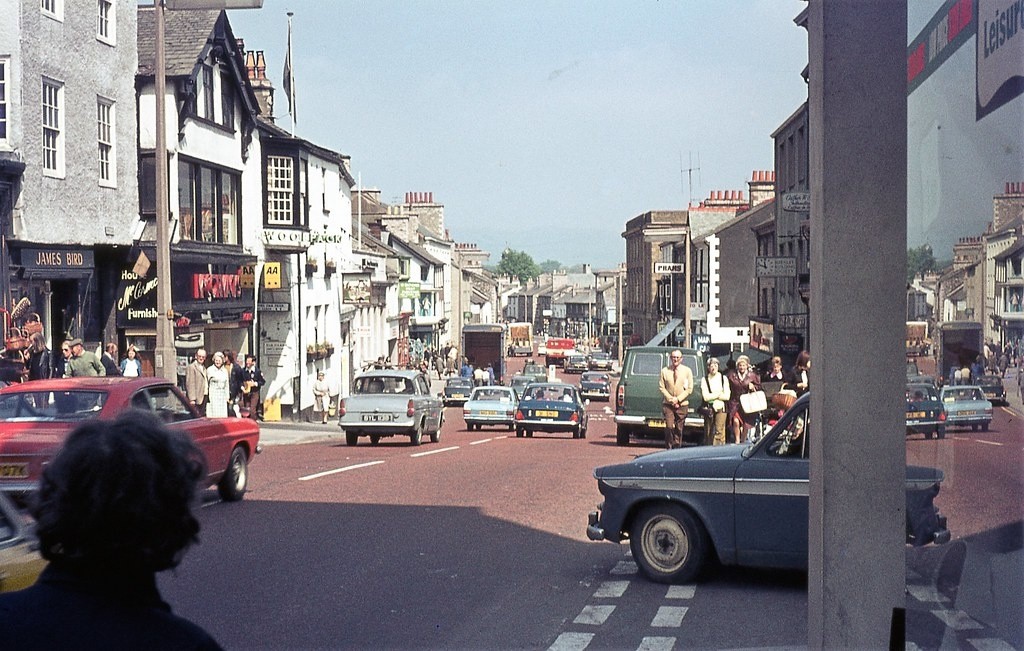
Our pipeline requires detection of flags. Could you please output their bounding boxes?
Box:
[282,23,297,125]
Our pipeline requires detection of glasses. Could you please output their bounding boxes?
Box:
[62,348,72,351]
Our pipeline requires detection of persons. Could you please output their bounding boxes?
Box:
[954,338,1024,406]
[29,331,141,418]
[0,409,222,651]
[188,346,262,452]
[313,371,329,423]
[661,349,812,449]
[365,340,493,396]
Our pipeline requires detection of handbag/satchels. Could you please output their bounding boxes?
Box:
[328,402,336,417]
[696,399,712,416]
[740,387,768,414]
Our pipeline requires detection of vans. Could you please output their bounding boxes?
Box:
[613,346,702,446]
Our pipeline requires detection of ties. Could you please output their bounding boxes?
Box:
[674,367,677,385]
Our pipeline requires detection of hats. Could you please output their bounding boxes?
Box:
[69,339,83,347]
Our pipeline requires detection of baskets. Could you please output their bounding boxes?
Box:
[772,384,798,409]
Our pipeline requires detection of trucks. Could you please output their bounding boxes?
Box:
[508,322,533,356]
[544,341,576,365]
[461,324,506,385]
[936,323,984,388]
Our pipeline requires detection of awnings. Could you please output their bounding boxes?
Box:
[646,318,683,346]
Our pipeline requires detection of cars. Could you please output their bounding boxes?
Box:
[444,364,612,438]
[0,376,259,503]
[0,489,50,594]
[536,343,546,356]
[563,352,611,373]
[339,370,443,447]
[587,392,811,585]
[907,361,1006,438]
